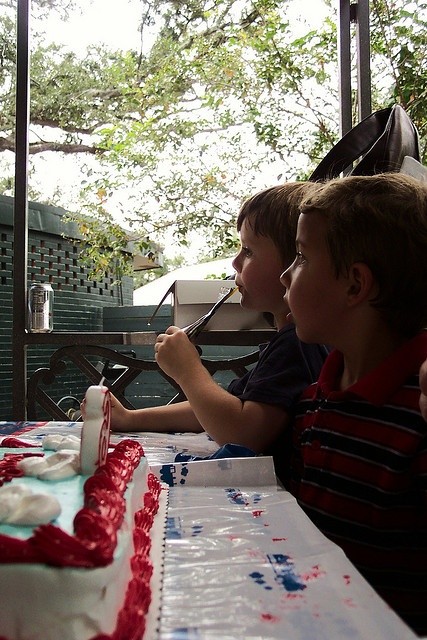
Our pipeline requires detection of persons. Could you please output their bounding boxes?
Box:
[280,173,427,635]
[80,180,329,480]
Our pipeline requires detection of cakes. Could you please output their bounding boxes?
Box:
[0,383,167,640]
